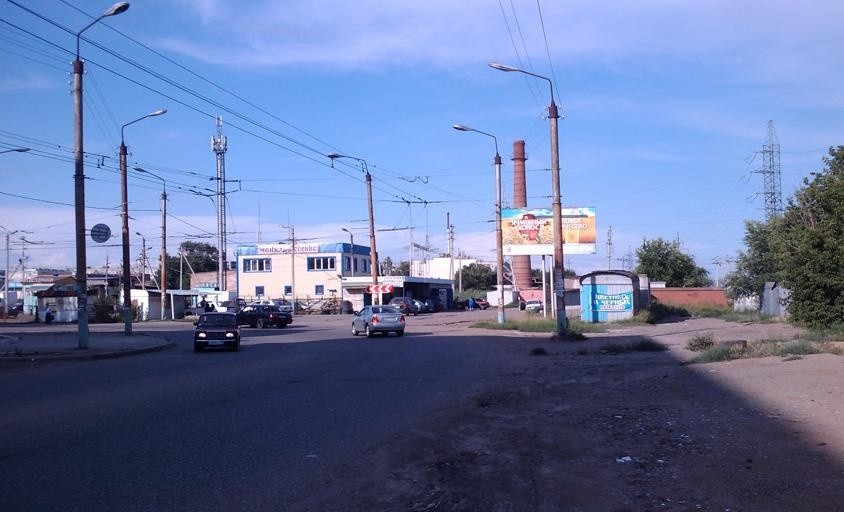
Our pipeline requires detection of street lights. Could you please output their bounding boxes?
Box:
[279,224,295,315]
[119,109,167,335]
[0,148,31,154]
[487,62,568,335]
[4,230,18,318]
[452,124,504,325]
[72,2,131,350]
[328,153,379,305]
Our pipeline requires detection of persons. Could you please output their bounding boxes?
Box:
[204,304,212,312]
[453,296,460,304]
[468,296,474,311]
[199,297,207,306]
[42,303,51,323]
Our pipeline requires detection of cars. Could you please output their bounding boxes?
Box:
[525,299,543,310]
[0,299,24,319]
[456,298,489,310]
[183,298,294,352]
[351,305,406,338]
[388,296,442,316]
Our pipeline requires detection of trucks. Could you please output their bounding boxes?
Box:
[0,291,17,308]
